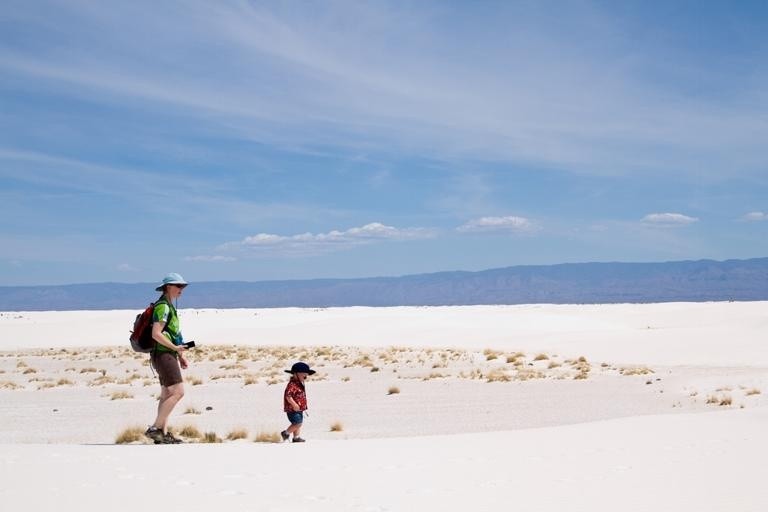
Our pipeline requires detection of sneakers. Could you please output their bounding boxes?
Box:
[144,424,183,444]
[281,430,290,442]
[292,436,305,442]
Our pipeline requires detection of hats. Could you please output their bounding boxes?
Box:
[283,361,316,376]
[154,272,189,293]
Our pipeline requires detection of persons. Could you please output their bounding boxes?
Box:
[143,270,190,446]
[279,363,315,443]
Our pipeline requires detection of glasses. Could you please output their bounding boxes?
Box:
[166,284,187,289]
